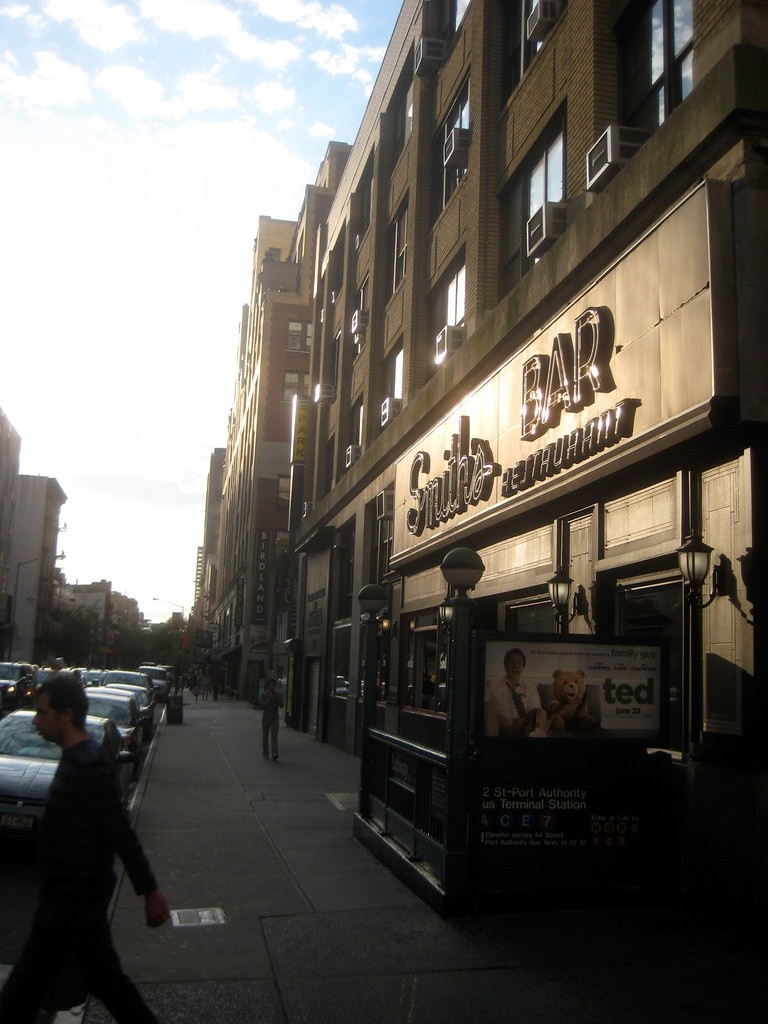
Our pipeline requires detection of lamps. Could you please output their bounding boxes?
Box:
[380,609,397,640]
[438,593,454,634]
[547,565,587,628]
[674,529,730,613]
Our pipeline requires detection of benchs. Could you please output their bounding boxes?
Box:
[129,700,154,740]
[146,675,163,703]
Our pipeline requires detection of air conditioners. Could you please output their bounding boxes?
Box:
[314,384,335,403]
[376,490,394,521]
[416,36,446,74]
[354,333,366,344]
[526,0,563,41]
[354,234,364,251]
[351,309,367,334]
[526,201,566,259]
[332,290,338,303]
[381,397,402,428]
[321,309,324,322]
[584,123,652,191]
[436,325,466,364]
[345,445,361,468]
[443,128,469,168]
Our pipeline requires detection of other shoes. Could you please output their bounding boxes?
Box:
[273,753,279,760]
[264,753,268,757]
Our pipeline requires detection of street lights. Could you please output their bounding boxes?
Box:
[152,594,187,625]
[6,554,67,660]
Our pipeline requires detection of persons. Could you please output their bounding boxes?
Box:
[187,667,225,702]
[490,647,547,738]
[257,679,285,760]
[0,682,169,1024]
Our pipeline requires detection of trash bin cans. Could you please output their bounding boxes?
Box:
[166,695,183,724]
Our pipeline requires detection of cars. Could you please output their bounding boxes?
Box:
[0,705,138,840]
[0,661,176,758]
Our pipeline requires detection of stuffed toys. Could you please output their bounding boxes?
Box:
[552,670,596,729]
